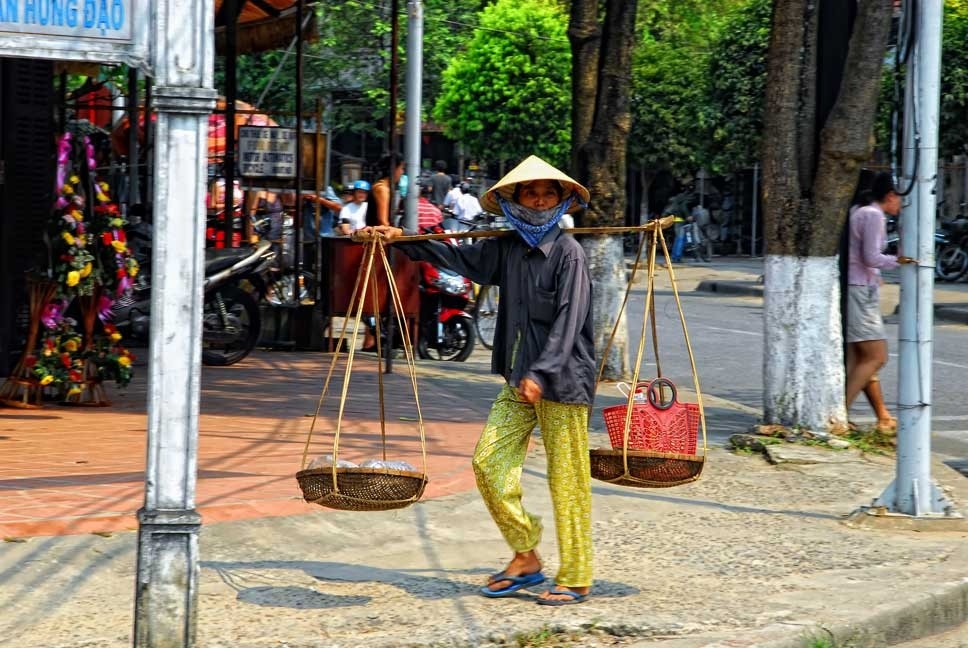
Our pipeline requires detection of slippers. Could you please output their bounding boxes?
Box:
[536,583,590,605]
[479,570,546,598]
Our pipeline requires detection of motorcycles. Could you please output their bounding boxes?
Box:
[88,215,284,370]
[869,211,968,278]
[406,215,480,370]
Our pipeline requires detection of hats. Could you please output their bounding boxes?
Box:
[479,154,590,217]
[455,181,470,189]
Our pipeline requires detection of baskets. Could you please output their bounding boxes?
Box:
[296,467,428,512]
[603,377,699,455]
[589,449,707,488]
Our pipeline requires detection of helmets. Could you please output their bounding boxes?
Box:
[354,179,371,191]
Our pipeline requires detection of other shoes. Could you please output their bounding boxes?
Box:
[876,416,898,437]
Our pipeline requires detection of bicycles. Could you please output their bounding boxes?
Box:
[679,205,713,264]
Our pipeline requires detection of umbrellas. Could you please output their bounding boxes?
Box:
[111,91,280,162]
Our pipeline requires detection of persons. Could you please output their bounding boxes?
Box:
[352,153,597,607]
[197,152,719,356]
[843,171,904,434]
